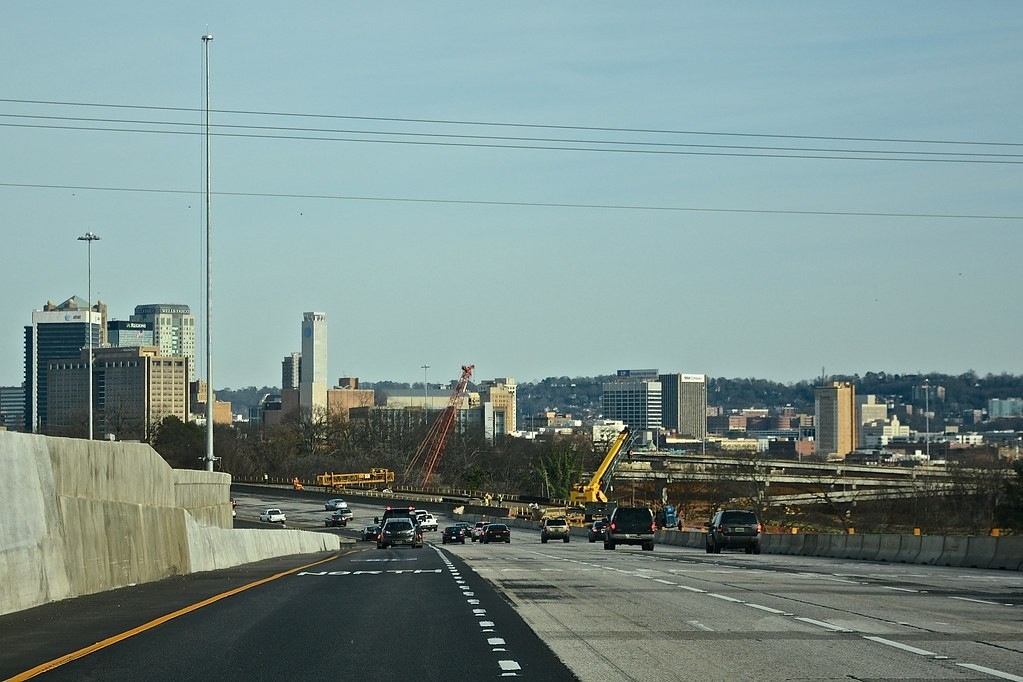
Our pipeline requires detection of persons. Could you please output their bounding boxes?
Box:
[532,500,539,520]
[263,472,268,485]
[294,476,300,490]
[482,492,502,508]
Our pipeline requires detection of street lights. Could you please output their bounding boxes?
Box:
[419,363,431,425]
[75,231,102,440]
[201,29,221,472]
[924,378,930,468]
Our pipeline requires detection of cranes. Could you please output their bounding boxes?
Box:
[398,363,476,493]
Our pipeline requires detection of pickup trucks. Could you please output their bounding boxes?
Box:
[259,509,287,524]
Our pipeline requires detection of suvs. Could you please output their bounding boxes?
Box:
[454,522,472,538]
[704,508,763,554]
[540,516,571,544]
[470,521,492,542]
[602,506,659,551]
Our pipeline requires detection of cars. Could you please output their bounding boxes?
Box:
[479,524,489,543]
[324,514,346,527]
[334,509,354,521]
[587,521,607,543]
[230,498,238,518]
[441,525,466,545]
[324,499,347,512]
[378,518,418,549]
[482,523,511,544]
[373,505,439,549]
[361,526,380,542]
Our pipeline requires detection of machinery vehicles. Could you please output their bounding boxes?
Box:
[539,425,641,529]
[315,468,395,493]
